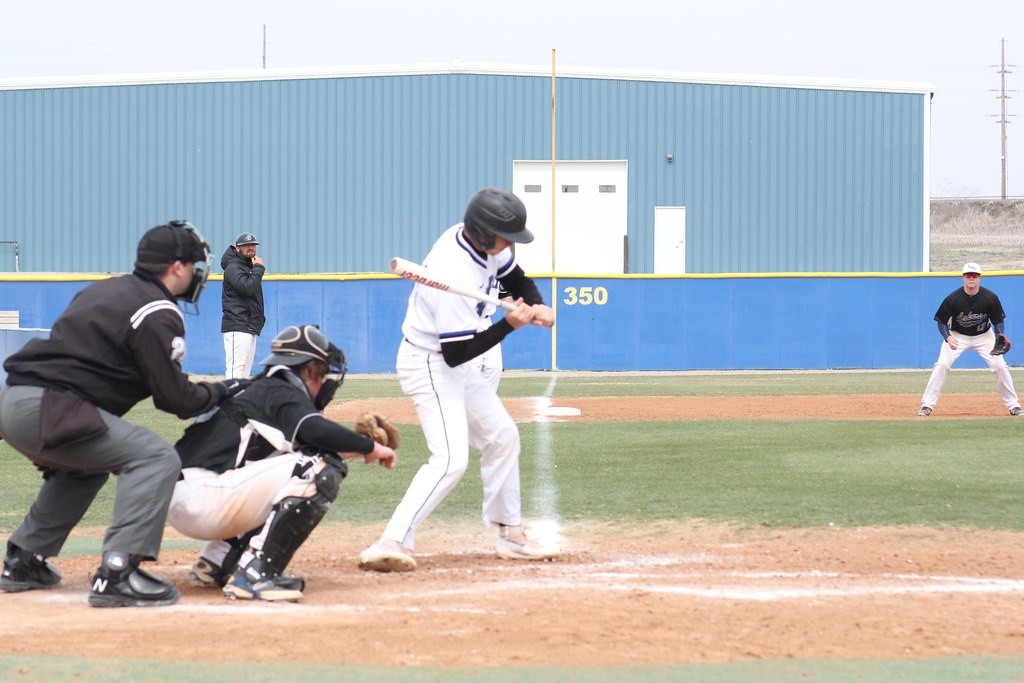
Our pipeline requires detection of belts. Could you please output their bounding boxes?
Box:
[176,471,184,481]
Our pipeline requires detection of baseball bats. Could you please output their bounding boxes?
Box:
[389,256,518,312]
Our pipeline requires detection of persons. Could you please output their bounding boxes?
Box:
[357,190,560,572]
[0,220,247,605]
[168,323,397,600]
[221,234,264,398]
[917,262,1024,416]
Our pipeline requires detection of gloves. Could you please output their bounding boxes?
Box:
[222,378,253,397]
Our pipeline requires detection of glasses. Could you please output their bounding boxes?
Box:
[963,273,980,278]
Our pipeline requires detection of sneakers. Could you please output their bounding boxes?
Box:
[918,407,932,416]
[189,558,233,587]
[0,545,61,590]
[356,539,416,572]
[222,570,306,602]
[88,563,181,608]
[495,533,564,560]
[1010,407,1024,415]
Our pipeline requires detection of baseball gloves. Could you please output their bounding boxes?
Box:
[990,332,1010,355]
[357,413,401,451]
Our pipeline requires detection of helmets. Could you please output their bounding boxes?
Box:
[463,188,534,249]
[236,233,259,246]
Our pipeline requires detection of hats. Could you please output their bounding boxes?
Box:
[962,262,982,274]
[136,219,207,272]
[259,324,321,366]
